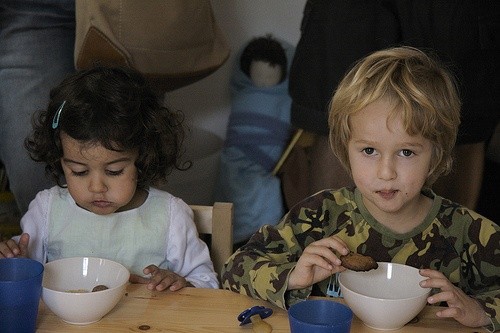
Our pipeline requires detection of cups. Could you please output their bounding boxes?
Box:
[288,299,353,333]
[0,257,45,333]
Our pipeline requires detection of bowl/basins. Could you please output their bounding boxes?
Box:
[338,262,432,331]
[40,256,131,326]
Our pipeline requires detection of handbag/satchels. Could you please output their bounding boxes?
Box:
[74,0,231,92]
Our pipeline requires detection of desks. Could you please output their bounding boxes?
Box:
[34,285,490,333]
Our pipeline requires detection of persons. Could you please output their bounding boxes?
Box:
[239,31,287,89]
[282,0,499,212]
[0,0,77,219]
[219,45,500,331]
[0,65,220,292]
